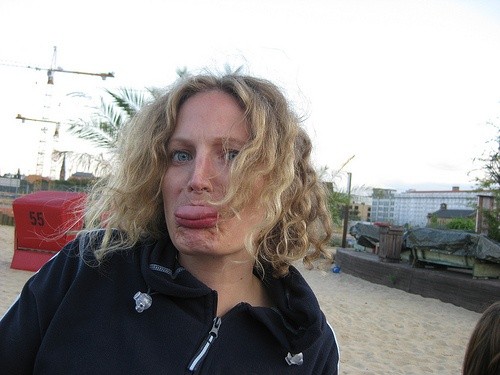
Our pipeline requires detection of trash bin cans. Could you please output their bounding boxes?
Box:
[379,226,403,263]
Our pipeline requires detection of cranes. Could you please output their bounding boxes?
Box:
[16,45,115,193]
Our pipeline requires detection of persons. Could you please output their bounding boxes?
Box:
[0,64,338,375]
